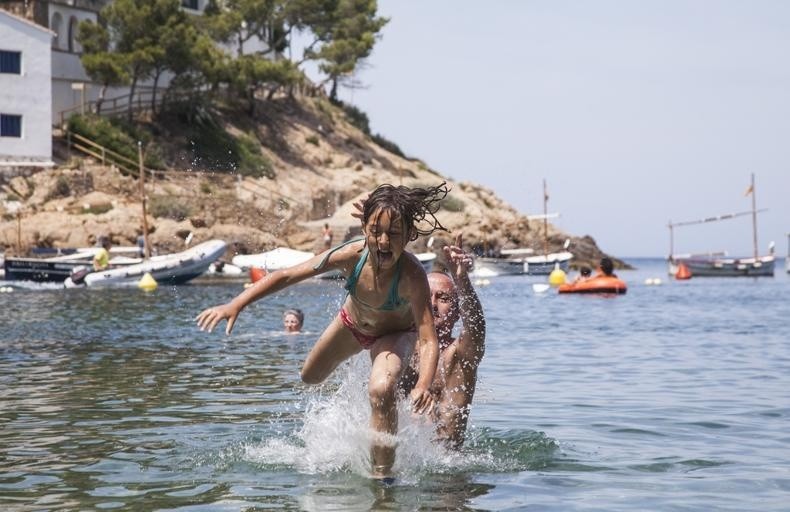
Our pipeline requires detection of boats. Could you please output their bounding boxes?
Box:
[558,275,627,294]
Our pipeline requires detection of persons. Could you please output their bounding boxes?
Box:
[84,234,96,247]
[92,241,111,272]
[319,223,331,251]
[593,257,616,277]
[29,230,42,247]
[136,225,158,259]
[574,267,591,283]
[40,235,53,248]
[193,179,450,489]
[282,308,304,333]
[397,245,486,455]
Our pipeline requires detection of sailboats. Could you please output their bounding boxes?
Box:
[665,171,790,277]
[477,177,575,276]
